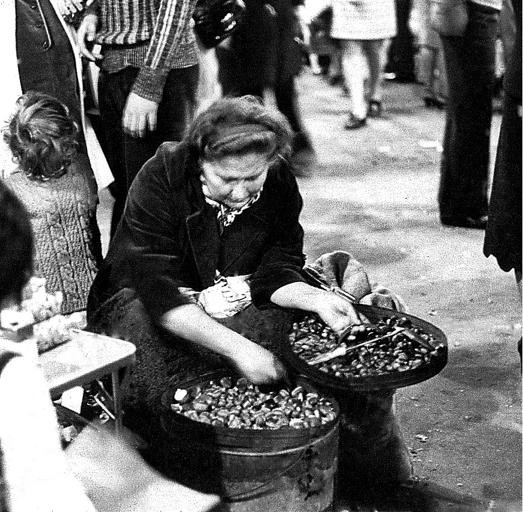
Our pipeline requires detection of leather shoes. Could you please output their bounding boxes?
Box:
[346,113,367,130]
[441,214,488,229]
[368,99,382,118]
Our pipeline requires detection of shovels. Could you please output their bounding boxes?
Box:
[305,326,435,366]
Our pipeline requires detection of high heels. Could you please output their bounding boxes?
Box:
[422,86,446,111]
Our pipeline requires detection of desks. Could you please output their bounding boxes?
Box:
[1,324,134,449]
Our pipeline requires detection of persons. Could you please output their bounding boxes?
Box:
[480,0,521,372]
[0,89,100,315]
[410,0,447,112]
[0,176,96,512]
[8,1,334,268]
[79,93,362,452]
[434,0,503,231]
[329,0,397,132]
